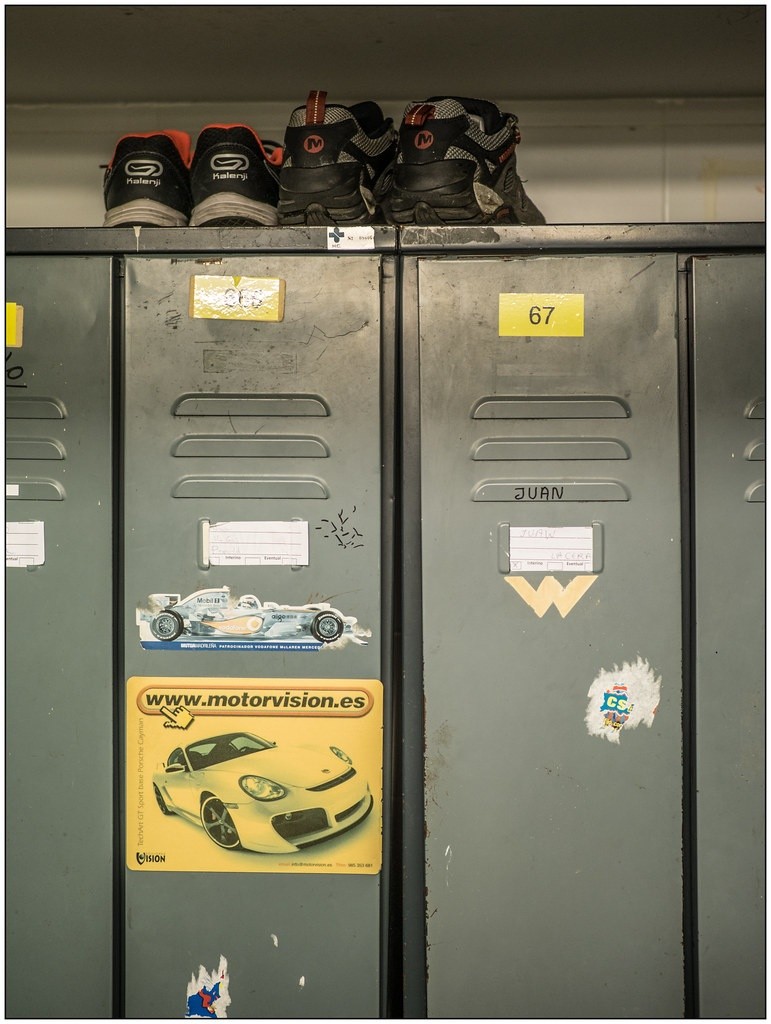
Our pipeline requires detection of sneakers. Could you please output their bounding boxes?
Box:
[277,89,400,225]
[189,123,283,226]
[381,95,546,226]
[98,128,194,227]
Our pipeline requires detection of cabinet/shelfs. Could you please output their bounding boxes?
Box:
[5,221,766,1019]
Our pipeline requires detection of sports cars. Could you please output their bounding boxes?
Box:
[149,729,374,857]
